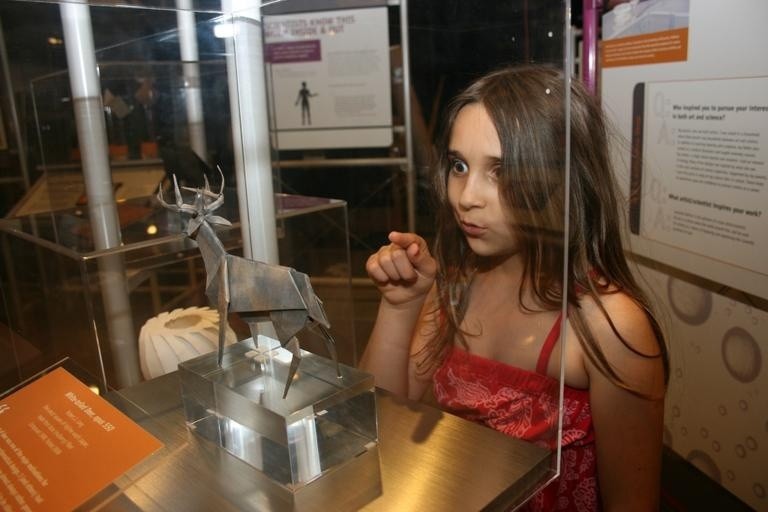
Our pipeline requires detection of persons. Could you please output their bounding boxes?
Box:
[356,62,671,512]
